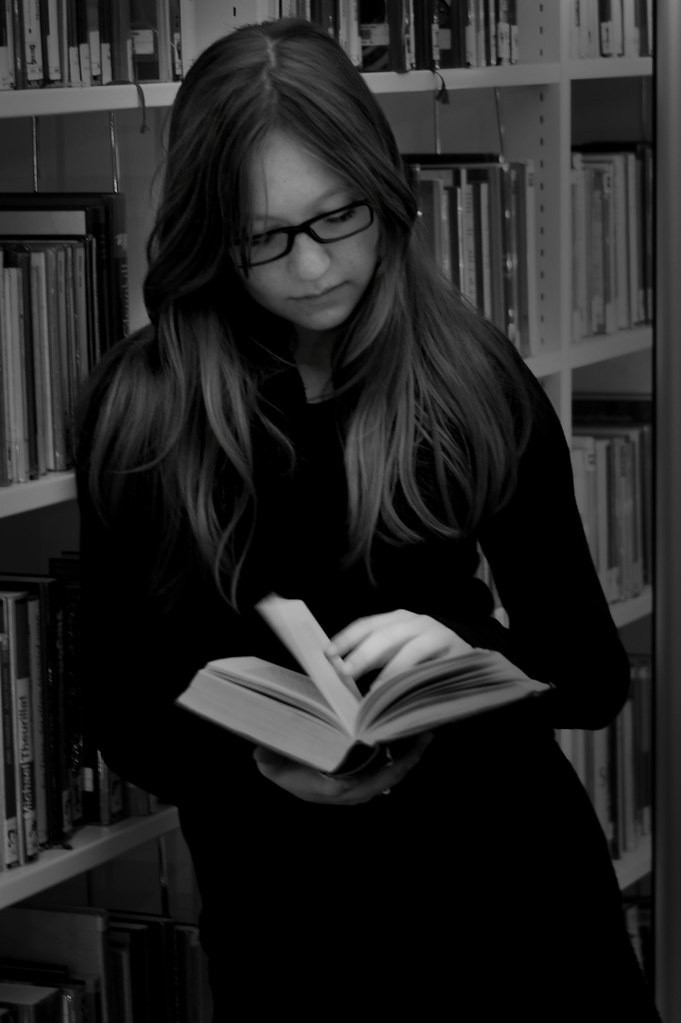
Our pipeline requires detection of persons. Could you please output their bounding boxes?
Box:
[54,21,661,1023]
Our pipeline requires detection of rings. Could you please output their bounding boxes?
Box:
[381,787,391,796]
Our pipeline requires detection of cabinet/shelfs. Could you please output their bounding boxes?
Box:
[0,0,661,1023]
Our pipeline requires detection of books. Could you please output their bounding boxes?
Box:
[571,388,657,605]
[0,900,207,1023]
[0,190,131,487]
[281,0,655,74]
[1,0,184,90]
[174,592,558,778]
[571,652,656,862]
[398,152,541,360]
[0,549,163,872]
[571,139,656,340]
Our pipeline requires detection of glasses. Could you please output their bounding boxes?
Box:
[222,197,375,267]
